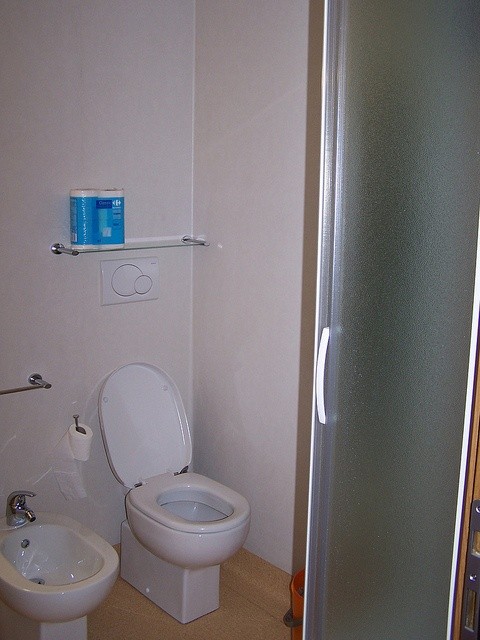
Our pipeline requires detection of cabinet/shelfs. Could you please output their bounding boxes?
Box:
[52,235,210,256]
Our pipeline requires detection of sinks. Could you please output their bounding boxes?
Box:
[0,524,104,586]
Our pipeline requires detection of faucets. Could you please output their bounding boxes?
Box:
[7,490,37,526]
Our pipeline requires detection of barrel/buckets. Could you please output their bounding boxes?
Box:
[283,568,306,640]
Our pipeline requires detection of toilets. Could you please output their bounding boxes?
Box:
[99,363,251,626]
